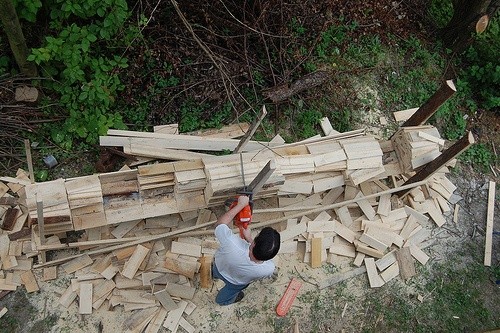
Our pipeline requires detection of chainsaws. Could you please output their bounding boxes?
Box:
[224,152,255,238]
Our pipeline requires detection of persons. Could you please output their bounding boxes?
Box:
[211,195,280,306]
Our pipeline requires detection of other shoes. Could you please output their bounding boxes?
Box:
[220,290,244,306]
